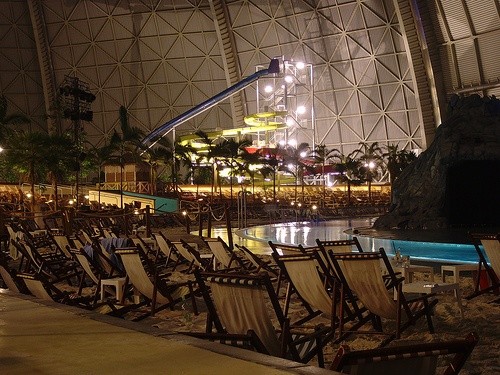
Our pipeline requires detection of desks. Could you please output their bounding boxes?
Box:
[392,264,434,282]
[98,276,140,303]
[199,254,232,270]
[441,265,488,298]
[173,243,198,251]
[392,281,463,322]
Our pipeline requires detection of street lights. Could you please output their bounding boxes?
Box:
[296,62,316,185]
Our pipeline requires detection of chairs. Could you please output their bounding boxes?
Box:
[0,191,500,375]
